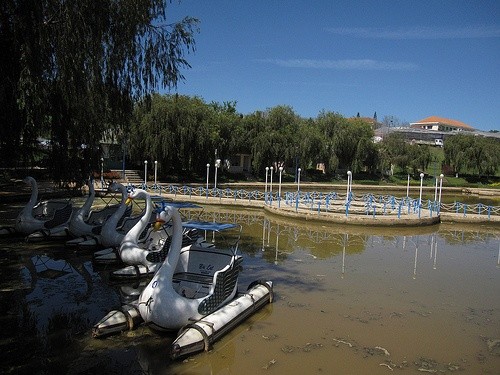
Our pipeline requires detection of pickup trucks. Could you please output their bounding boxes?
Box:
[430,138,444,148]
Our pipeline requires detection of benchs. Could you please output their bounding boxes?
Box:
[34,214,53,221]
[172,272,214,295]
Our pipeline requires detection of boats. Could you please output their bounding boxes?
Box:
[0,175,275,362]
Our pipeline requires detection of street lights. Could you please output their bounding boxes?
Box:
[270,167,274,192]
[100,158,104,181]
[297,168,302,191]
[279,166,283,197]
[215,164,218,188]
[346,171,351,198]
[144,160,148,185]
[419,173,425,203]
[206,163,211,189]
[154,160,158,185]
[265,167,269,193]
[439,173,444,204]
